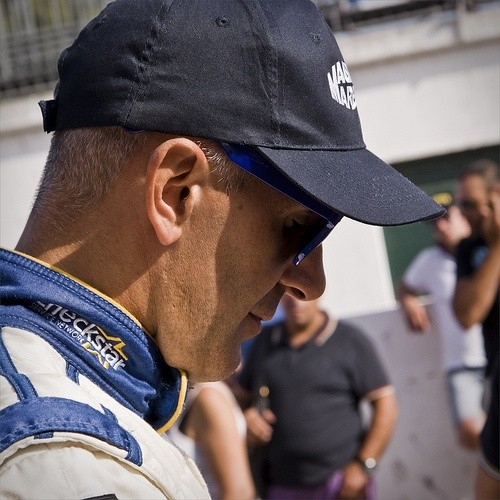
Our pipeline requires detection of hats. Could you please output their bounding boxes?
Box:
[431,192,454,217]
[38,0,445,227]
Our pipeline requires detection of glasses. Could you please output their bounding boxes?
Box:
[217,137,342,264]
